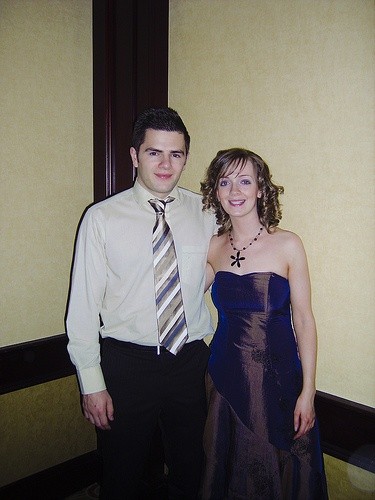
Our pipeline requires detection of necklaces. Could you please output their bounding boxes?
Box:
[229,225,265,267]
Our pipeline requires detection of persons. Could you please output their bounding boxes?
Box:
[66,107,225,500]
[200,148,329,500]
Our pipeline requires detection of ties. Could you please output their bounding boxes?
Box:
[148,198,189,358]
